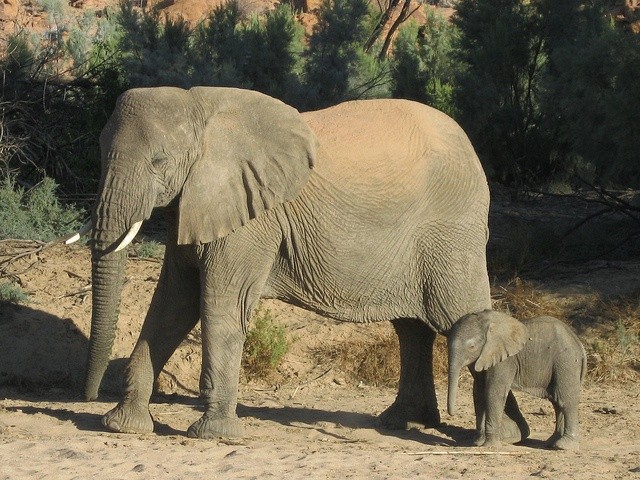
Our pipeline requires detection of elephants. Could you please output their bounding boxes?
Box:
[64,84,531,447]
[447,308,588,451]
